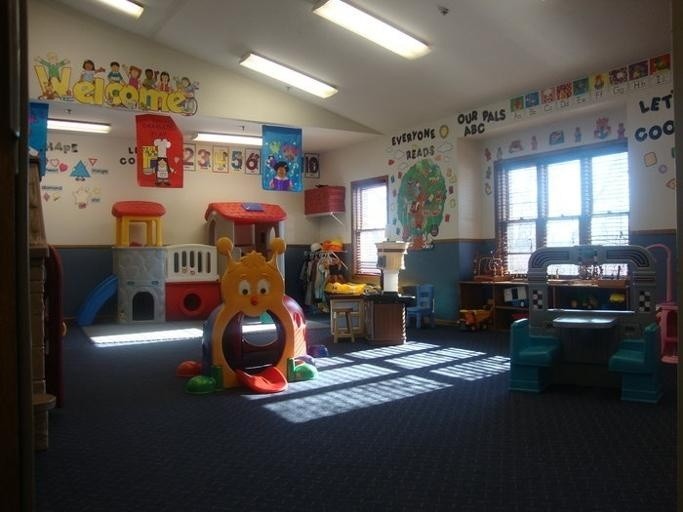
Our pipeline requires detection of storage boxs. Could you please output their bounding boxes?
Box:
[305,185,345,214]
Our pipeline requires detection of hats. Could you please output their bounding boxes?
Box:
[274,161,289,172]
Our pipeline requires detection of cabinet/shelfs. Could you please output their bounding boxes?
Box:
[458,277,633,334]
[330,294,365,338]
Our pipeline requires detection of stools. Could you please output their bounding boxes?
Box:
[331,307,355,344]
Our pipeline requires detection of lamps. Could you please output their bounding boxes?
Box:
[308,0,431,62]
[101,0,144,19]
[238,52,337,99]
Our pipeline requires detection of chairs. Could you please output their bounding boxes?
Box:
[607,322,662,404]
[507,318,560,393]
[406,284,433,329]
